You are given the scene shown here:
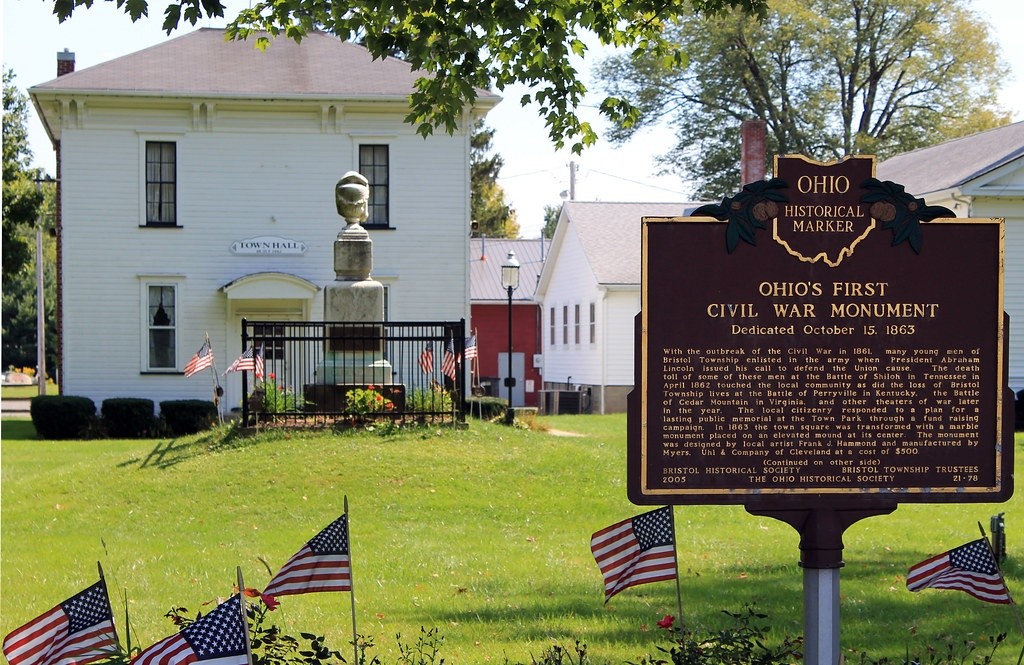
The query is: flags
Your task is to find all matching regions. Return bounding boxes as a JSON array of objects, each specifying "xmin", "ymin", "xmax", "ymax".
[
  {"xmin": 255, "ymin": 343, "xmax": 264, "ymax": 382},
  {"xmin": 224, "ymin": 346, "xmax": 254, "ymax": 374},
  {"xmin": 263, "ymin": 515, "xmax": 352, "ymax": 595},
  {"xmin": 3, "ymin": 579, "xmax": 116, "ymax": 665},
  {"xmin": 441, "ymin": 338, "xmax": 455, "ymax": 381},
  {"xmin": 184, "ymin": 341, "xmax": 214, "ymax": 378},
  {"xmin": 128, "ymin": 593, "xmax": 250, "ymax": 665},
  {"xmin": 590, "ymin": 505, "xmax": 677, "ymax": 605},
  {"xmin": 418, "ymin": 341, "xmax": 433, "ymax": 374},
  {"xmin": 457, "ymin": 335, "xmax": 478, "ymax": 363},
  {"xmin": 906, "ymin": 538, "xmax": 1015, "ymax": 603}
]
